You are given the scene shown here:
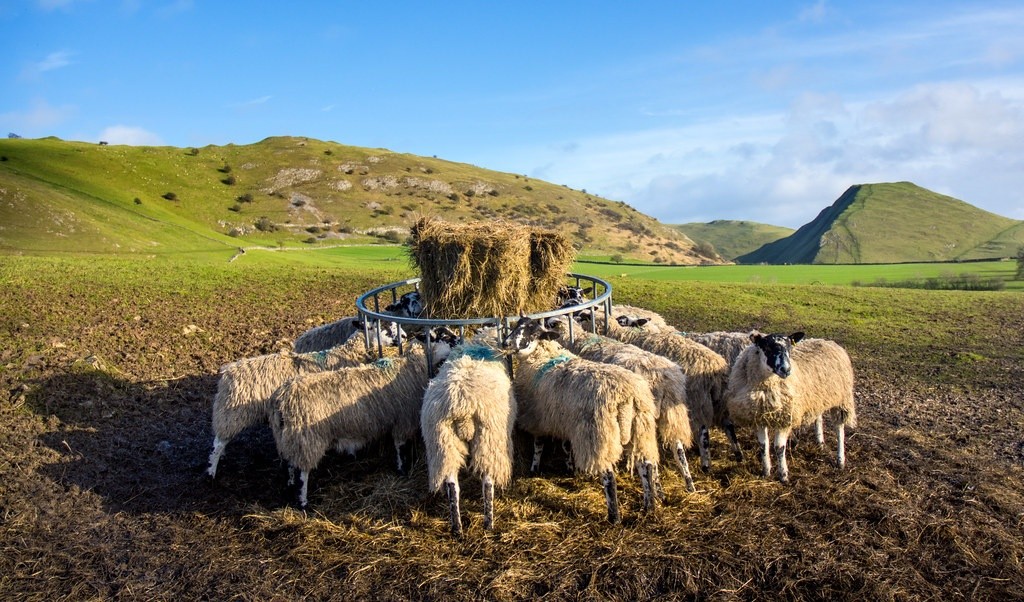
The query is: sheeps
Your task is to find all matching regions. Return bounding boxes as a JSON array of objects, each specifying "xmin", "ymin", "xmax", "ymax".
[{"xmin": 204, "ymin": 288, "xmax": 859, "ymax": 540}]
